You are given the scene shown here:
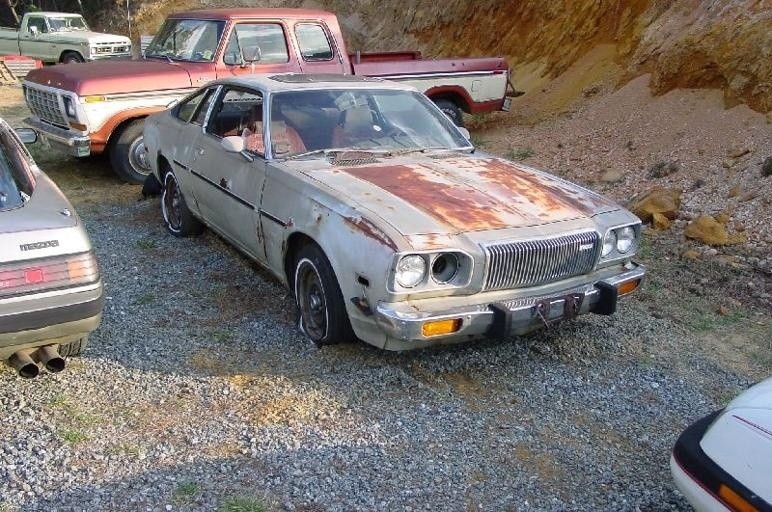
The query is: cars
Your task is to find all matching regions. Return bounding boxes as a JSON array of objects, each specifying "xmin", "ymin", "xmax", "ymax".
[
  {"xmin": 669, "ymin": 377, "xmax": 772, "ymax": 512},
  {"xmin": 142, "ymin": 73, "xmax": 646, "ymax": 351},
  {"xmin": 0, "ymin": 118, "xmax": 103, "ymax": 378}
]
